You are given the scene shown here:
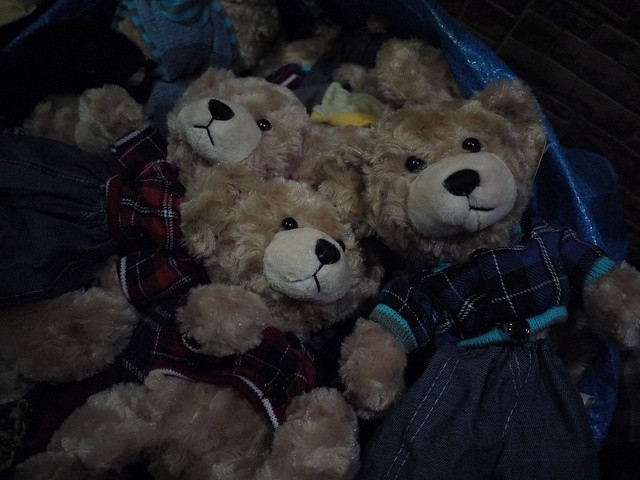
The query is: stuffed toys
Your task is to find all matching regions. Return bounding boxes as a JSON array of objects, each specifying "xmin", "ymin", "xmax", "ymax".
[
  {"xmin": 104, "ymin": 1, "xmax": 342, "ymax": 85},
  {"xmin": 10, "ymin": 163, "xmax": 406, "ymax": 479},
  {"xmin": 302, "ymin": 36, "xmax": 453, "ymax": 123},
  {"xmin": 0, "ymin": 67, "xmax": 333, "ymax": 404},
  {"xmin": 337, "ymin": 77, "xmax": 640, "ymax": 479}
]
